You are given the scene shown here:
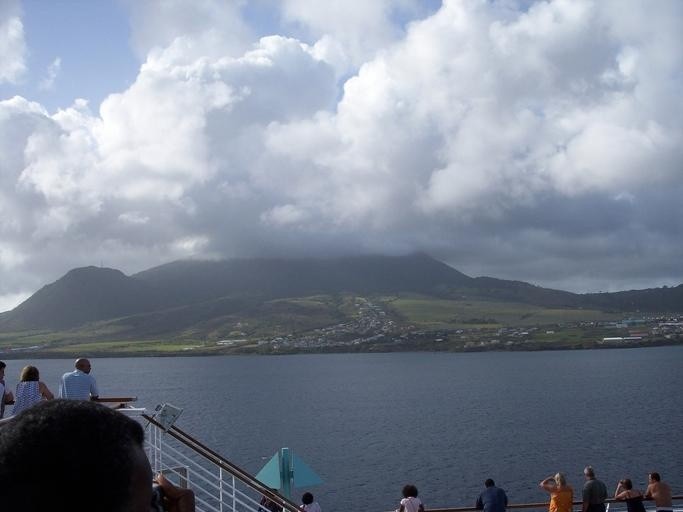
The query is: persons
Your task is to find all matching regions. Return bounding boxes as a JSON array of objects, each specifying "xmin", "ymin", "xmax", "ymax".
[
  {"xmin": 539, "ymin": 472, "xmax": 573, "ymax": 512},
  {"xmin": 645, "ymin": 472, "xmax": 673, "ymax": 512},
  {"xmin": 614, "ymin": 479, "xmax": 646, "ymax": 512},
  {"xmin": 0, "ymin": 361, "xmax": 14, "ymax": 420},
  {"xmin": 476, "ymin": 478, "xmax": 508, "ymax": 512},
  {"xmin": 257, "ymin": 489, "xmax": 283, "ymax": 512},
  {"xmin": 399, "ymin": 484, "xmax": 424, "ymax": 512},
  {"xmin": 58, "ymin": 358, "xmax": 98, "ymax": 401},
  {"xmin": 1, "ymin": 398, "xmax": 196, "ymax": 512},
  {"xmin": 298, "ymin": 492, "xmax": 321, "ymax": 512},
  {"xmin": 582, "ymin": 465, "xmax": 608, "ymax": 512},
  {"xmin": 12, "ymin": 365, "xmax": 54, "ymax": 416}
]
